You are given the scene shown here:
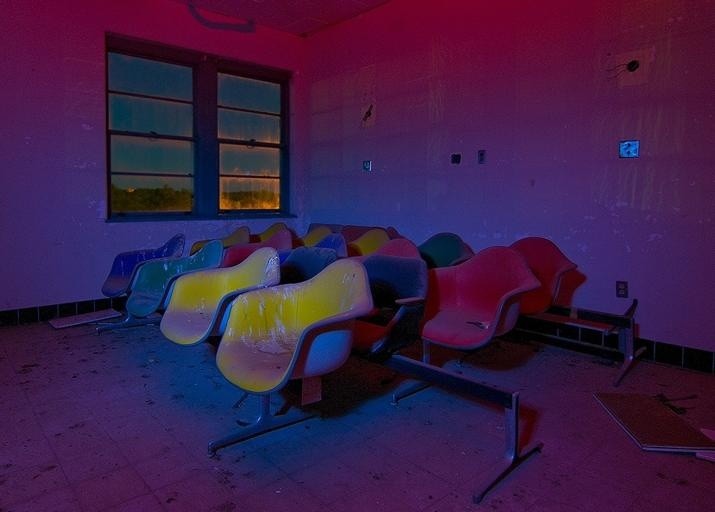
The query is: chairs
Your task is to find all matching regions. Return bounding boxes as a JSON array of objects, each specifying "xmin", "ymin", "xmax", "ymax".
[{"xmin": 96, "ymin": 221, "xmax": 655, "ymax": 507}]
